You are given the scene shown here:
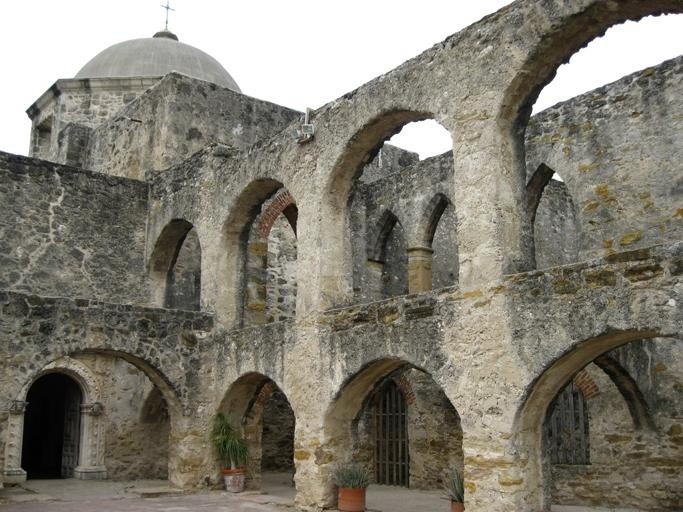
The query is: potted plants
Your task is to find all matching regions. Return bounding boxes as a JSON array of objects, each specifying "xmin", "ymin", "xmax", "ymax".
[
  {"xmin": 210, "ymin": 411, "xmax": 247, "ymax": 493},
  {"xmin": 438, "ymin": 469, "xmax": 465, "ymax": 512},
  {"xmin": 333, "ymin": 463, "xmax": 373, "ymax": 512}
]
